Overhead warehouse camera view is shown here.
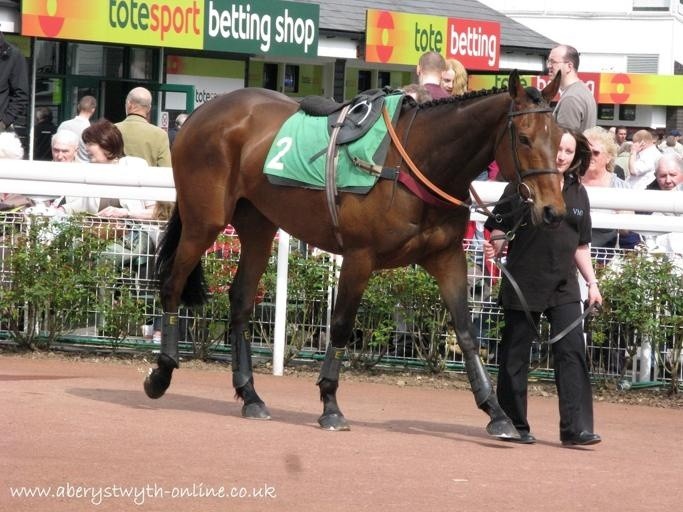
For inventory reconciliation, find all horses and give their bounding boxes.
[142,69,568,441]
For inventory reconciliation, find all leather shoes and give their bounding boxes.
[501,431,535,443]
[562,430,601,446]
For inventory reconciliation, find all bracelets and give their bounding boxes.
[585,279,601,288]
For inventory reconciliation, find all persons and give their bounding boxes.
[407,49,452,103]
[0,34,30,131]
[442,58,468,94]
[587,120,683,274]
[466,159,502,300]
[544,43,598,130]
[1,86,188,334]
[482,126,602,446]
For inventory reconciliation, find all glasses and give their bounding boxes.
[545,60,568,66]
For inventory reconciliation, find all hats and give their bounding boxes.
[671,129,681,137]
